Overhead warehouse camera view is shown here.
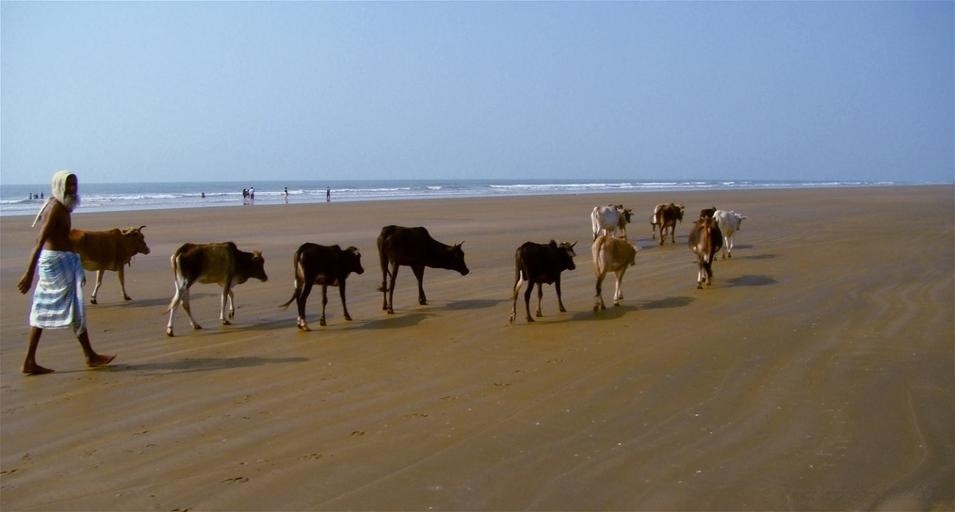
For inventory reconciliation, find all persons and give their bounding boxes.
[201,191,205,200]
[284,186,288,198]
[242,186,255,200]
[29,191,45,200]
[12,167,118,376]
[326,185,330,201]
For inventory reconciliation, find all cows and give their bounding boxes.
[688,207,748,290]
[591,235,639,313]
[509,240,578,322]
[590,204,635,242]
[161,242,268,337]
[278,242,365,332]
[68,225,151,305]
[649,203,685,246]
[376,225,469,315]
